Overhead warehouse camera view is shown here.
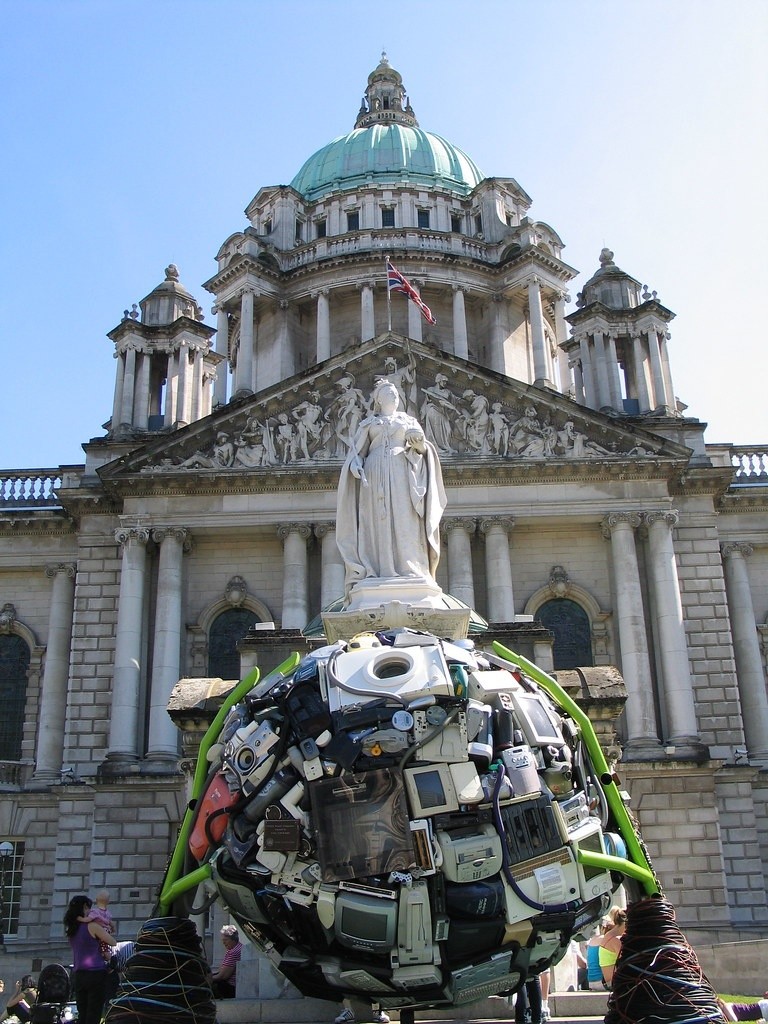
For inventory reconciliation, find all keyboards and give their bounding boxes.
[340,970,412,1009]
[453,972,520,1004]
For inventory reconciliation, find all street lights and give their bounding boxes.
[0,842,15,945]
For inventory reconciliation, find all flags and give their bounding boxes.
[387,261,436,326]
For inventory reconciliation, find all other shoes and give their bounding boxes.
[542,1007,551,1020]
[523,1007,533,1023]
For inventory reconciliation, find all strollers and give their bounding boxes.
[29,964,72,1024]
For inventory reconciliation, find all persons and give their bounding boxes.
[336,377,447,592]
[78,890,116,956]
[169,337,618,469]
[210,924,243,999]
[63,895,117,1024]
[514,904,627,1024]
[0,974,38,1024]
[717,998,768,1024]
[335,999,390,1024]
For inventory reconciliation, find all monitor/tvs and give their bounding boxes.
[508,692,565,748]
[403,763,459,820]
[309,766,419,884]
[574,740,587,799]
[214,877,269,924]
[258,889,329,956]
[335,892,398,954]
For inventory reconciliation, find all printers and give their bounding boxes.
[466,698,492,773]
[435,823,503,883]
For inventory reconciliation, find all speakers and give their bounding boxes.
[500,744,542,798]
[225,719,280,787]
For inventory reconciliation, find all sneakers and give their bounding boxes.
[335,1009,355,1023]
[372,1011,390,1023]
